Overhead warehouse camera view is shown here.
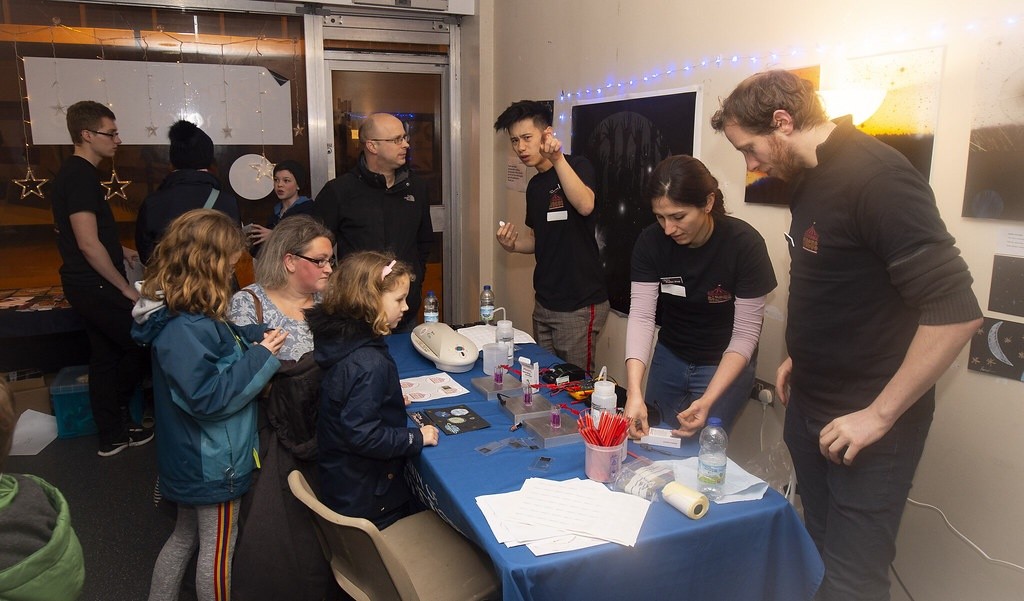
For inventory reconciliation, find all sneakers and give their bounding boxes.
[97,427,154,457]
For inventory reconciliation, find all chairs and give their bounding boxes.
[288,470,502,601]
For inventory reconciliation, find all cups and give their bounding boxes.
[482,342,509,376]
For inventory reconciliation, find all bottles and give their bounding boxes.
[696,416,729,502]
[480,285,495,326]
[590,380,617,430]
[423,290,439,323]
[495,320,515,367]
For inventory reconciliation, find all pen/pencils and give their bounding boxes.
[577,408,634,447]
[628,451,672,476]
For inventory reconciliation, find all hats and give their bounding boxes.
[168,120,215,171]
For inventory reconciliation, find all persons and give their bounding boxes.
[303,250,439,531]
[131,209,289,601]
[52,101,155,456]
[314,113,432,334]
[245,160,314,259]
[622,155,778,444]
[711,70,984,601]
[0,375,86,601]
[134,120,240,309]
[494,100,610,378]
[227,213,335,363]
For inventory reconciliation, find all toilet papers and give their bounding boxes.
[660,481,710,521]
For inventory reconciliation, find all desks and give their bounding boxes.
[381,322,826,601]
[0,287,86,337]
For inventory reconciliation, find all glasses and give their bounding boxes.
[292,253,334,267]
[85,128,119,140]
[371,133,410,145]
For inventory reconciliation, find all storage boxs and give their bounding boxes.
[3,364,145,440]
